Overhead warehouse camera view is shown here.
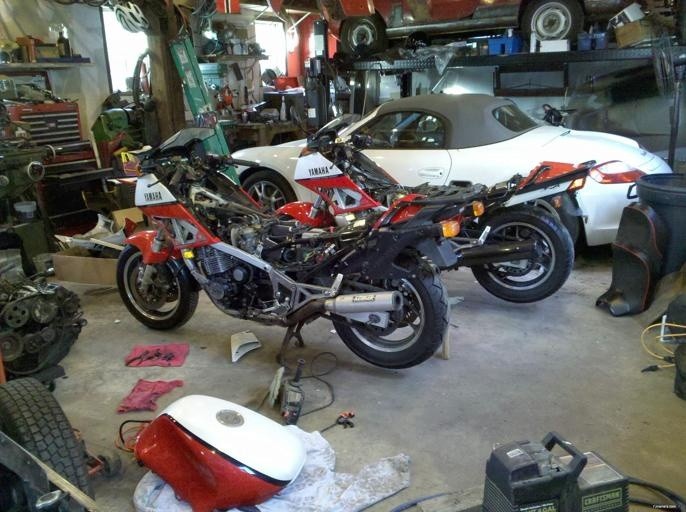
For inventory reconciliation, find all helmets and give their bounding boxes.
[406,31,431,49]
[114,0,150,33]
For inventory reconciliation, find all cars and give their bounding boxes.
[314,0,584,54]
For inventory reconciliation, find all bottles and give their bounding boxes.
[280,96,288,122]
[56,31,67,59]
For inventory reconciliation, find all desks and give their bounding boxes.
[219,117,307,154]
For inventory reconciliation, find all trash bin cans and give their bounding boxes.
[627,173,686,280]
